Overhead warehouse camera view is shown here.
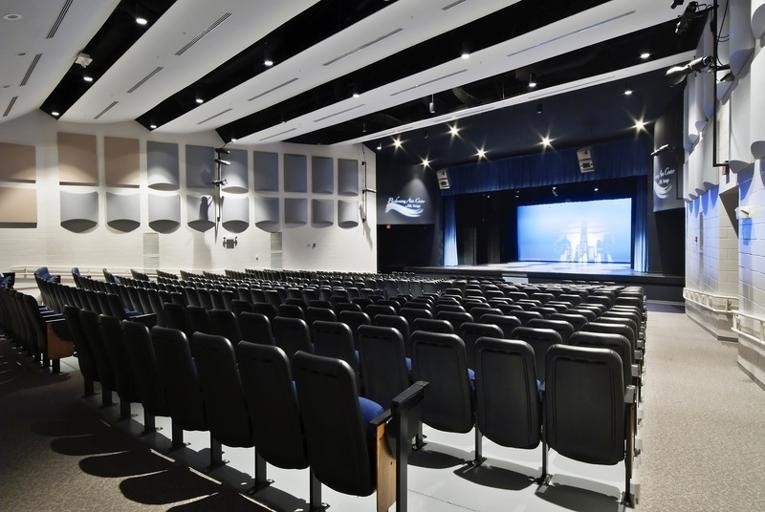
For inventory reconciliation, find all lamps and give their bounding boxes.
[212,148,230,187]
[651,142,676,159]
[665,55,713,88]
[671,0,713,36]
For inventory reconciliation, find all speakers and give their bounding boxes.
[578,143,604,173]
[437,170,454,188]
